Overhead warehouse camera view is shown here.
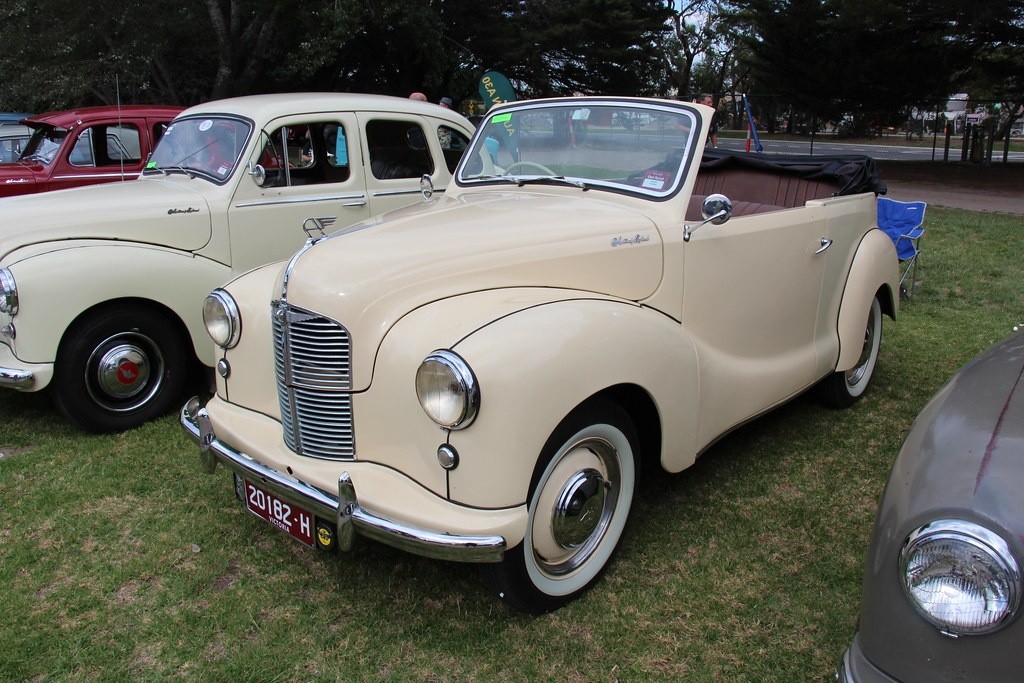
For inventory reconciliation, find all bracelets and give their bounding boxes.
[714,147,717,148]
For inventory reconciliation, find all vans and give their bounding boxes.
[927,93,973,135]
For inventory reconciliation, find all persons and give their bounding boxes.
[302,130,312,166]
[409,93,428,150]
[438,97,453,148]
[678,93,718,149]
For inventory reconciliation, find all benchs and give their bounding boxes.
[694,171,842,211]
[686,193,788,220]
[369,152,413,182]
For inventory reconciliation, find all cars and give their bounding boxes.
[1,120,142,164]
[838,328,1024,683]
[1010,129,1024,136]
[0,105,294,199]
[0,94,514,435]
[180,91,900,616]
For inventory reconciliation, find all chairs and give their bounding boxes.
[877,198,927,294]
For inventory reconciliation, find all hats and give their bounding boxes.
[440,97,452,107]
